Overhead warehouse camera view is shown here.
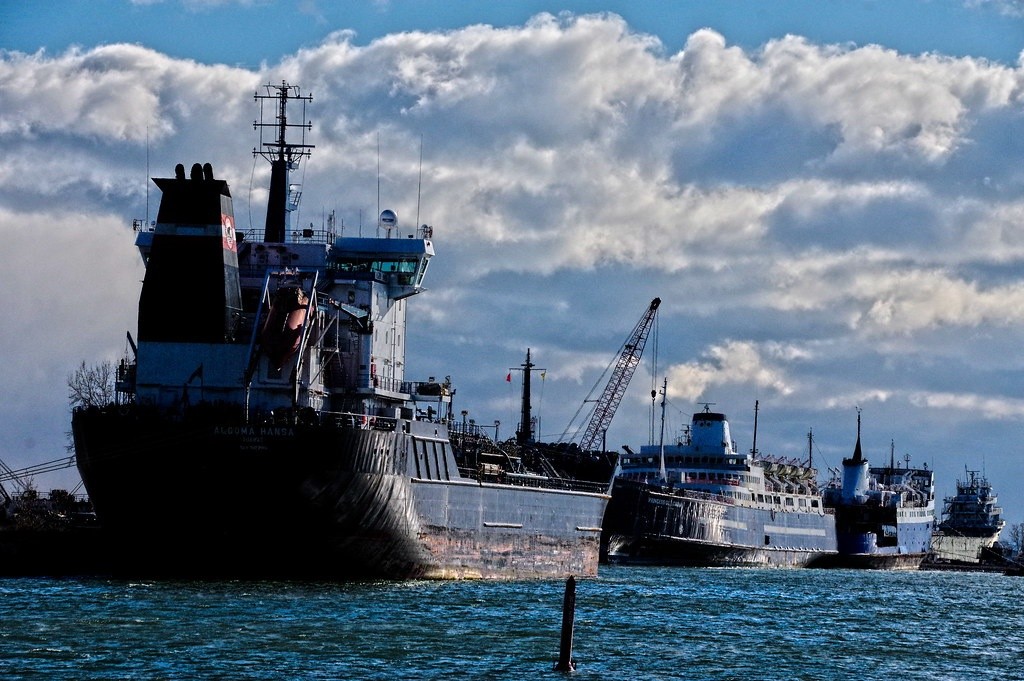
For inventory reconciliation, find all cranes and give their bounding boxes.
[563,294,659,474]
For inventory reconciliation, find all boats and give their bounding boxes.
[978,522,1024,574]
[926,462,1007,567]
[68,83,626,582]
[612,377,841,568]
[819,406,937,569]
